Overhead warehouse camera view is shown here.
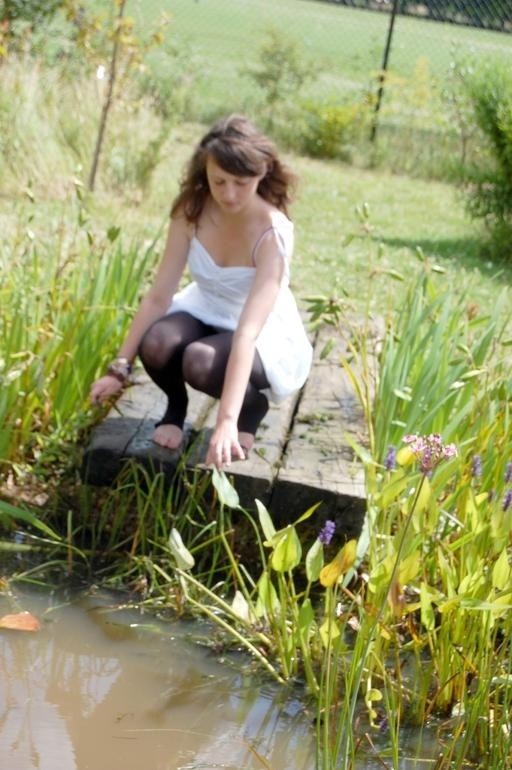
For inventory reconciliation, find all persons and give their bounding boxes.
[87,115,314,473]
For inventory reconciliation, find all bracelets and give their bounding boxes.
[102,354,134,380]
